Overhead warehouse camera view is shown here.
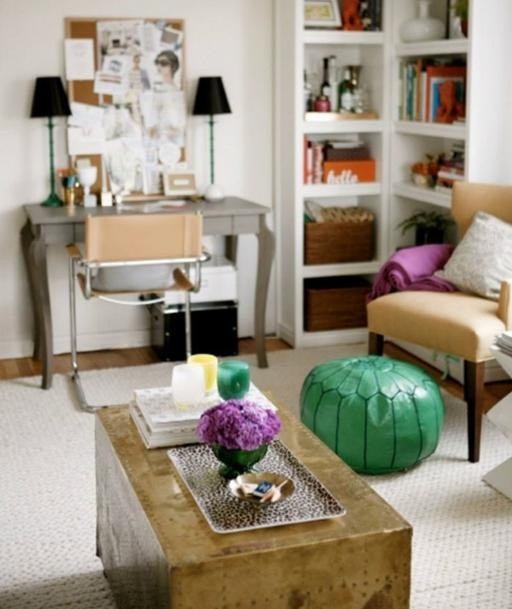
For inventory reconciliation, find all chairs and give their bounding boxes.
[67,207,203,378]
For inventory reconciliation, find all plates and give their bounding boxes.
[166,439,348,534]
[228,470,296,505]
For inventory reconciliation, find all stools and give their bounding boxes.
[301,355,446,474]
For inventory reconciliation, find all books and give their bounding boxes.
[128,379,278,449]
[400,57,466,124]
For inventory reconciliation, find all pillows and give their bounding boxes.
[431,211,511,300]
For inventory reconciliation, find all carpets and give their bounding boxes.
[0,350,512,609]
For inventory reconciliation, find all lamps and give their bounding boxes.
[30,76,73,208]
[190,77,233,200]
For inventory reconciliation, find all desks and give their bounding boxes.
[21,201,275,390]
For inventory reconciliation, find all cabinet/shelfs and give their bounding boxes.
[387,0,511,264]
[274,0,386,348]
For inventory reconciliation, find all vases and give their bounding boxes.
[211,442,270,479]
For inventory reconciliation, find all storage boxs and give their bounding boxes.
[305,219,375,265]
[323,157,375,183]
[304,280,371,332]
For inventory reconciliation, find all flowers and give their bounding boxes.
[199,401,282,452]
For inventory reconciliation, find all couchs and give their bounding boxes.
[368,179,511,465]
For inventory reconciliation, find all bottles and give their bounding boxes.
[319,57,331,111]
[338,69,354,116]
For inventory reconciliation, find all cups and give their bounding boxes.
[172,364,205,409]
[217,359,251,402]
[187,353,219,391]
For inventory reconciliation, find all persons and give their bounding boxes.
[127,54,150,90]
[436,81,465,123]
[154,50,179,90]
